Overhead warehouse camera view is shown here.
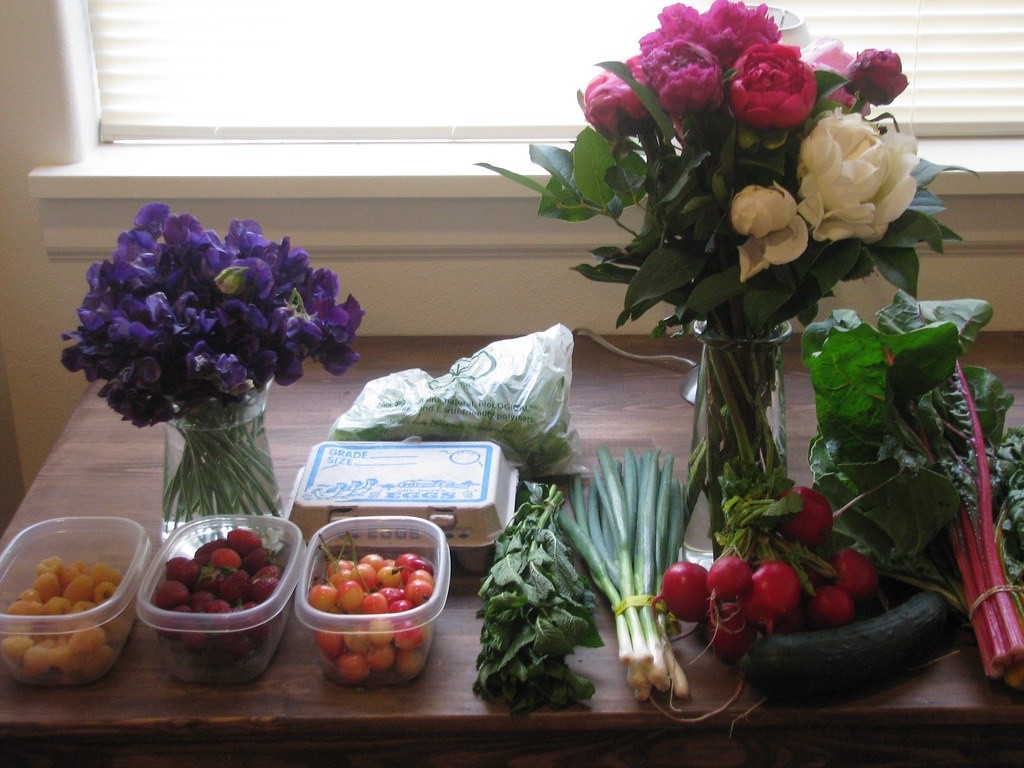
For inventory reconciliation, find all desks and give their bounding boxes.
[0,333,1023,768]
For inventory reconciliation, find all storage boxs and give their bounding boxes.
[0,515,152,688]
[137,516,306,684]
[294,514,451,685]
[289,441,520,570]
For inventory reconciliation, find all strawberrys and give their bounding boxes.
[151,528,285,669]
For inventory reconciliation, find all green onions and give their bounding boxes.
[558,446,691,701]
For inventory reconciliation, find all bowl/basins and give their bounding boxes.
[136,513,309,687]
[294,515,451,688]
[0,516,152,688]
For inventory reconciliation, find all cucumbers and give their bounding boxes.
[735,588,954,694]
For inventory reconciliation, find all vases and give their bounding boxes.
[162,374,277,544]
[677,319,793,568]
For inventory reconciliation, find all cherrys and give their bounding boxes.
[312,530,434,685]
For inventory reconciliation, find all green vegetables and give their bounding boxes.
[334,342,575,476]
[795,290,1024,690]
[472,478,605,726]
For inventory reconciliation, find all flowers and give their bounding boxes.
[60,201,365,520]
[477,0,980,567]
